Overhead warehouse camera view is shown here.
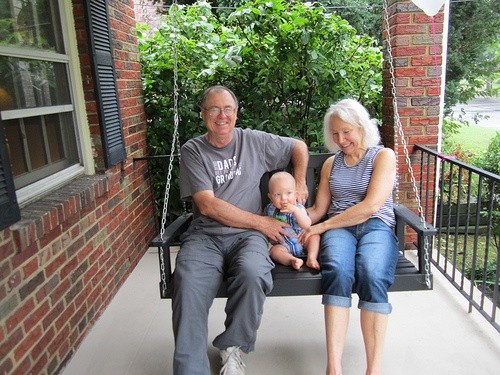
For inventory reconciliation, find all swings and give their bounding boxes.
[147,0,438,299]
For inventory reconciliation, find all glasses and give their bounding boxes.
[199,105,236,117]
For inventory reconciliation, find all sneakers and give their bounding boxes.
[217,345,246,374]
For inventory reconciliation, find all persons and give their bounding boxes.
[262,172,320,270]
[171,85,308,375]
[296,98,399,375]
